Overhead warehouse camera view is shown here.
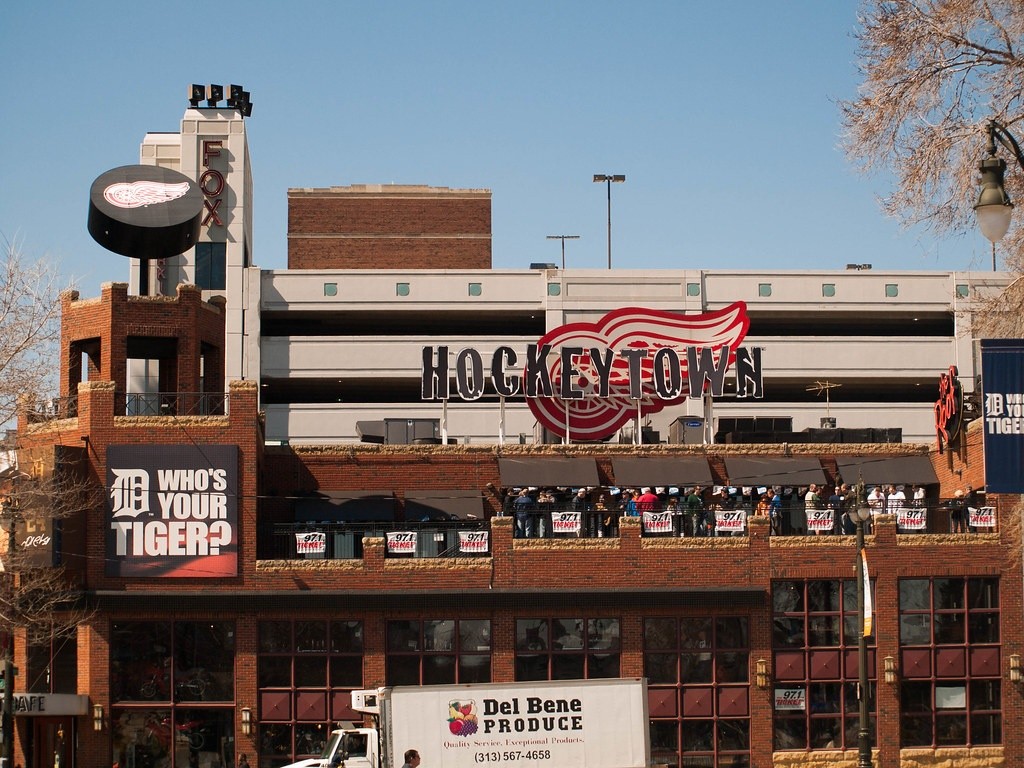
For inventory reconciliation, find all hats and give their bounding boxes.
[721,487,729,494]
[772,485,782,495]
[966,485,973,491]
[621,489,629,493]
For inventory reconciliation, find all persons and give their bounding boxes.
[402,749,421,768]
[504,483,978,535]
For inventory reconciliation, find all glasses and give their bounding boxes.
[813,488,816,490]
[875,489,881,492]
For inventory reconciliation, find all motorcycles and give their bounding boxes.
[141,657,207,700]
[141,713,207,750]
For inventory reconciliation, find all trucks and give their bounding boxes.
[277,676,653,768]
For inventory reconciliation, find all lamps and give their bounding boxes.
[884,653,895,684]
[756,656,766,687]
[240,707,252,735]
[1010,652,1021,682]
[94,703,103,731]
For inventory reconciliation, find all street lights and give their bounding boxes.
[593,173,626,270]
[546,234,580,268]
[843,480,873,768]
